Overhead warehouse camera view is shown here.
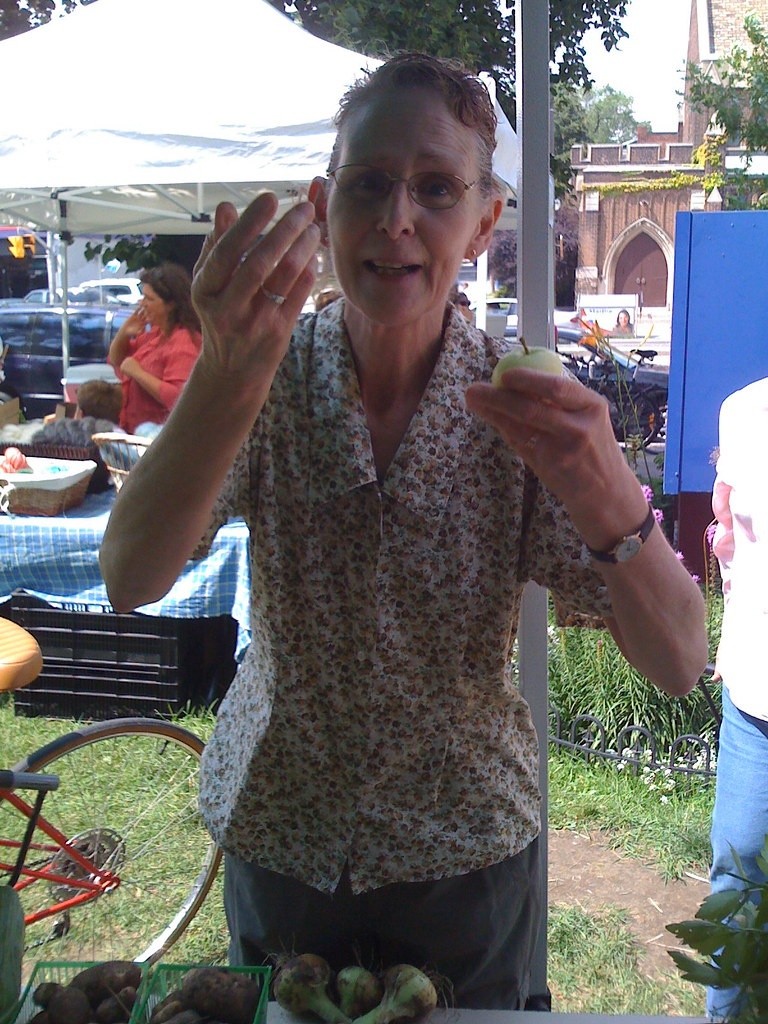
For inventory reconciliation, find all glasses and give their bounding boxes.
[457,301,471,306]
[327,162,484,210]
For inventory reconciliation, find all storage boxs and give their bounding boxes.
[10,961,272,1024]
[10,591,204,722]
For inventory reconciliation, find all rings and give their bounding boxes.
[260,282,286,305]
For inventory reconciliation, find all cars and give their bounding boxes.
[23,278,145,304]
[505,329,669,441]
[0,304,139,421]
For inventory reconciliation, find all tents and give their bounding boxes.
[0,0,555,376]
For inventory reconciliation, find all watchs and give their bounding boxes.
[586,504,655,565]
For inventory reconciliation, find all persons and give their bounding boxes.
[692,377,768,1018]
[108,262,203,433]
[613,310,633,334]
[450,281,476,326]
[99,52,709,1012]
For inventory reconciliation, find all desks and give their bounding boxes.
[0,488,251,670]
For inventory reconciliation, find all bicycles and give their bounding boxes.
[555,348,663,451]
[0,617,223,967]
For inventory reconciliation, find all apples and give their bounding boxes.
[490,335,562,386]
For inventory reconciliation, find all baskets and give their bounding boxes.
[12,960,149,1023]
[135,963,272,1024]
[0,442,110,479]
[92,432,156,493]
[0,456,97,517]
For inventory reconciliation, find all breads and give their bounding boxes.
[0,415,114,448]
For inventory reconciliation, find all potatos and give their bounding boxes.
[22,959,263,1024]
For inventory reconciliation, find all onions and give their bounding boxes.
[269,949,439,1024]
